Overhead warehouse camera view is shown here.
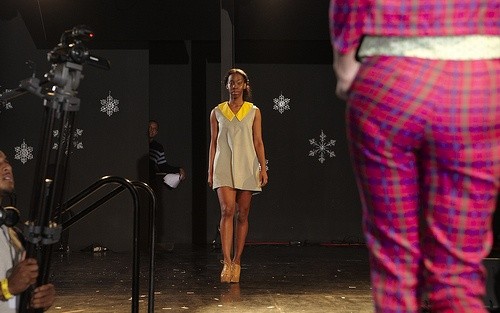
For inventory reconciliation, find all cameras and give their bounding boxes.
[48,28,110,67]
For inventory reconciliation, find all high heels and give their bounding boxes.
[220,263,231,282]
[230,263,241,282]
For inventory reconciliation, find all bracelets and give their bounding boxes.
[1,277,14,300]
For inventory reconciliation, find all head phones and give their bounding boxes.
[0,206,20,228]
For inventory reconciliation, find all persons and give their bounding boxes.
[148,120,187,249]
[0,150,57,313]
[208,68,269,283]
[330,0,500,313]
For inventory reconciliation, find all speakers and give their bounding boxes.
[480,257,500,313]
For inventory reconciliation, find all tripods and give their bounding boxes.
[0,62,81,313]
